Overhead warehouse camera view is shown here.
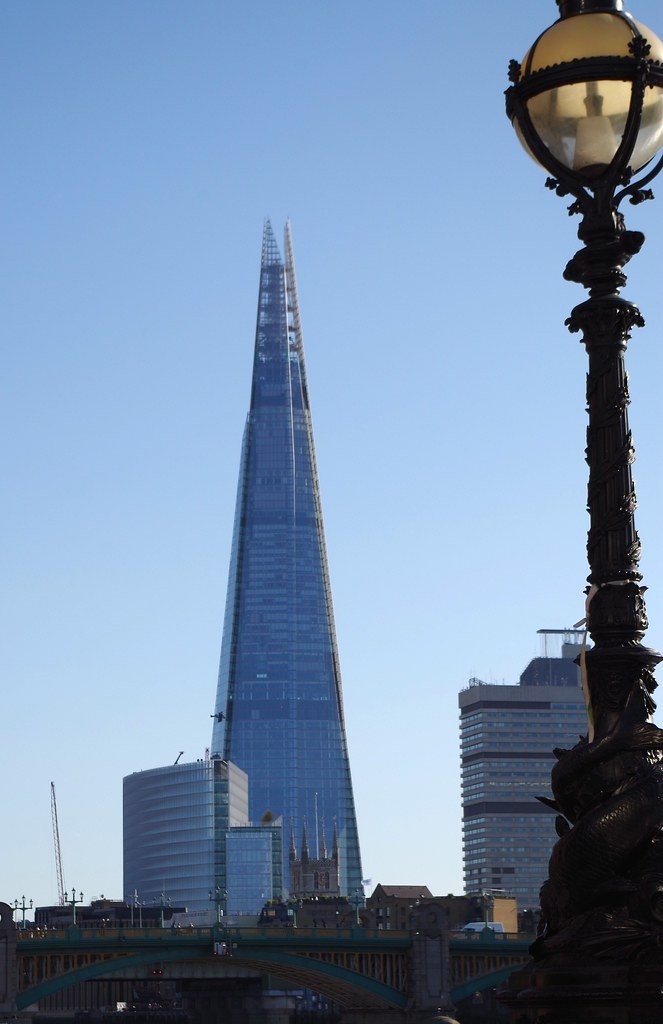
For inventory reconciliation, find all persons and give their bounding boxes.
[122,1004,130,1012]
[336,923,339,929]
[99,919,111,936]
[171,921,195,936]
[287,921,297,937]
[29,925,56,939]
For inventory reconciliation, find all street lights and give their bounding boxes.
[208,886,228,922]
[502,1,663,1015]
[14,894,33,931]
[64,887,84,925]
[153,893,172,928]
